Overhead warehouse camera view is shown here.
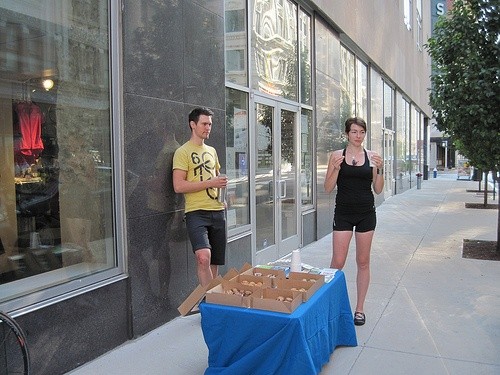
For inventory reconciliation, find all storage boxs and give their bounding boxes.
[269,278,317,302]
[285,272,325,290]
[253,288,303,314]
[177,278,264,317]
[232,262,286,288]
[216,267,272,289]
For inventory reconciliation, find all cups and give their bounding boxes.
[291,250,301,271]
[219,174,227,203]
[369,151,378,167]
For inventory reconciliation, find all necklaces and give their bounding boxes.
[348,147,361,159]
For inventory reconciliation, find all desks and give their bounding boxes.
[198,265,359,375]
[15,176,49,251]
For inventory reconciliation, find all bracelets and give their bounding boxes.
[377,167,384,175]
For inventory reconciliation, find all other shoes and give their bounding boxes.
[135,294,171,316]
[354,311,365,325]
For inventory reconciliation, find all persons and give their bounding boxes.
[325,117,384,325]
[173,106,228,288]
